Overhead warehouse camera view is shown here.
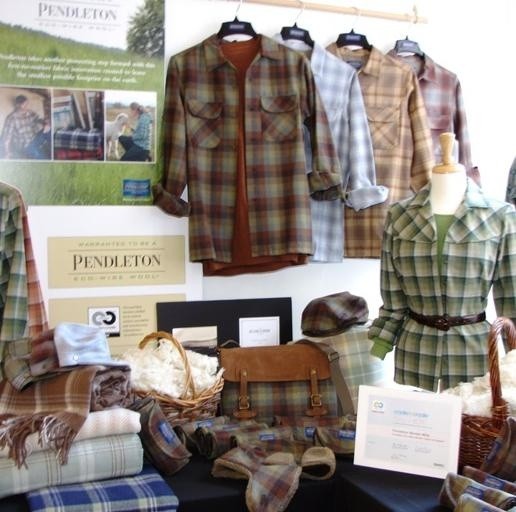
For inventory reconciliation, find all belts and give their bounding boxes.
[407,309,486,331]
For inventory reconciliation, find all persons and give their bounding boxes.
[3,91,49,158]
[366,162,516,395]
[118,100,153,162]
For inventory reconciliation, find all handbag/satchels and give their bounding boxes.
[220,346,338,420]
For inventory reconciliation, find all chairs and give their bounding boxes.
[156,296,294,353]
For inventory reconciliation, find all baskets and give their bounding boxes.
[458,317,516,471]
[132,333,225,426]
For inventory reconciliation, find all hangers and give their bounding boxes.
[325,4,382,60]
[212,0,265,44]
[269,0,320,57]
[387,13,428,63]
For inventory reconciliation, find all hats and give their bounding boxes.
[211,439,336,511]
[302,292,369,337]
